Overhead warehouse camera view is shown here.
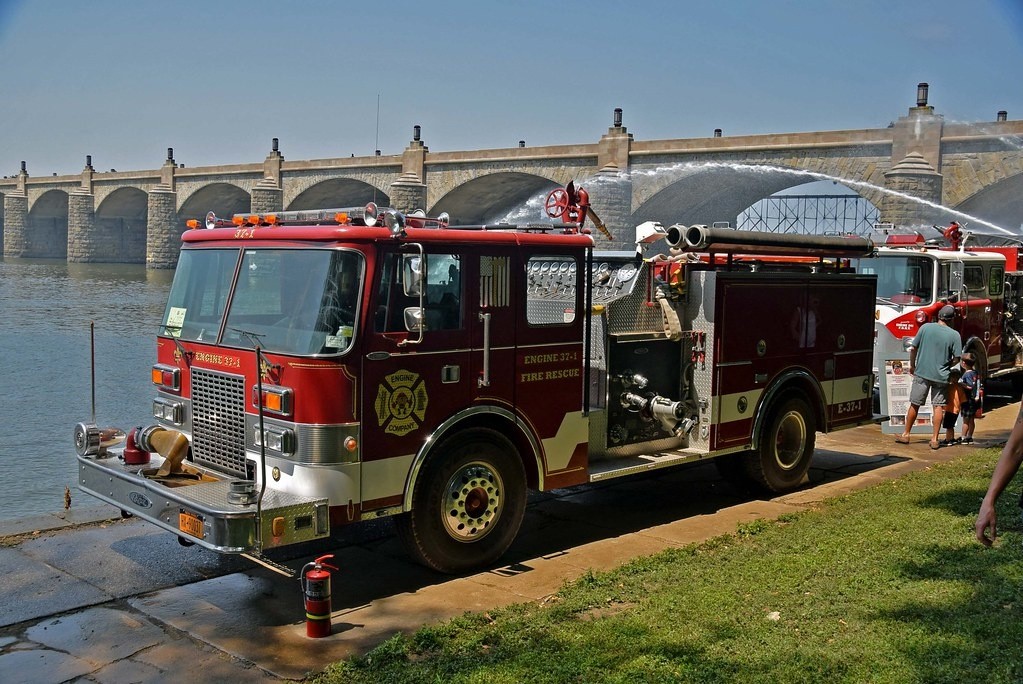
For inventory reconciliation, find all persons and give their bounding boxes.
[956,353,980,445]
[894,305,962,448]
[975,395,1023,547]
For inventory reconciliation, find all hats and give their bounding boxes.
[938,305,955,320]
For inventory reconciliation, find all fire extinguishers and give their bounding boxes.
[300,554,340,639]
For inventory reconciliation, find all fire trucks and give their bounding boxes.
[73,175,877,577]
[834,224,1023,409]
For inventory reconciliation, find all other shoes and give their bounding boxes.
[939,439,952,445]
[895,435,910,443]
[951,439,957,444]
[967,439,974,444]
[929,441,939,449]
[956,437,968,444]
